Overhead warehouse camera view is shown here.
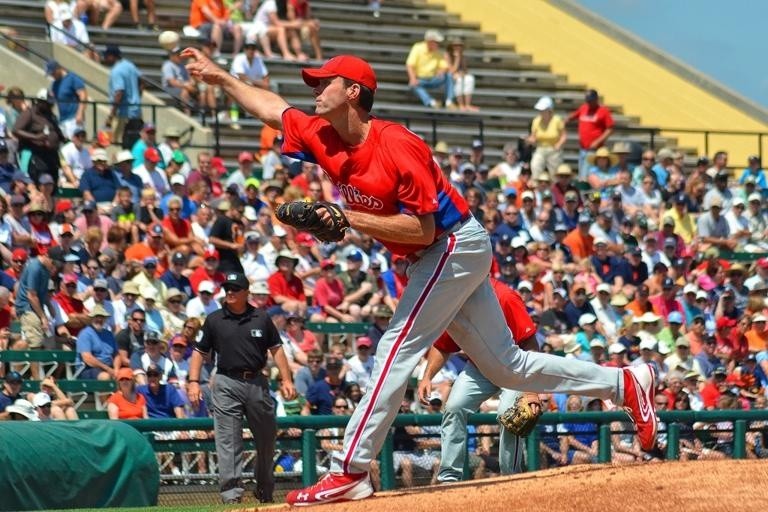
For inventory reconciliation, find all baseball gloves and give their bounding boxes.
[275,201,350,242]
[499,392,543,437]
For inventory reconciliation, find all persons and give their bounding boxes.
[1,0,768,508]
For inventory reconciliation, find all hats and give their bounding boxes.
[533,97,554,110]
[170,39,257,54]
[585,90,597,102]
[36,88,57,105]
[46,60,57,75]
[302,56,377,91]
[424,29,444,42]
[0,123,407,422]
[427,138,768,410]
[103,46,121,57]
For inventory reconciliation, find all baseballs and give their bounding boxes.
[159,30,179,49]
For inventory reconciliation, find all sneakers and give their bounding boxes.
[286,472,375,507]
[621,364,659,450]
